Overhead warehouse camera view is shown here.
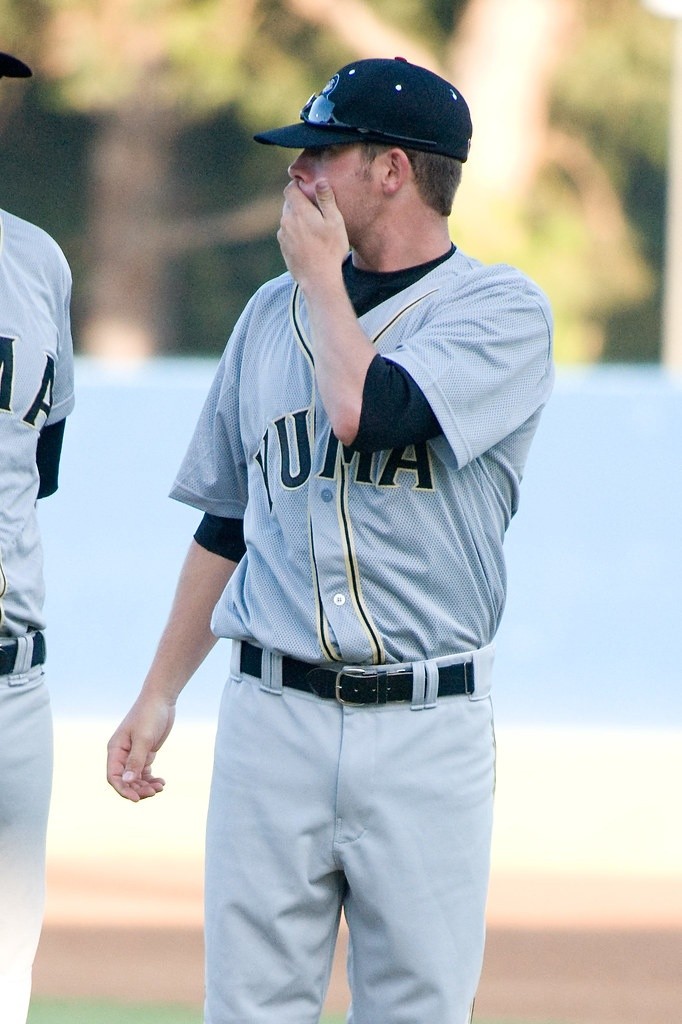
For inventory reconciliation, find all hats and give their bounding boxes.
[253,57,472,163]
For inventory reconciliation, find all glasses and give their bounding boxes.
[300,92,436,146]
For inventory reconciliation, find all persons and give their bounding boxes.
[107,56,554,1024]
[0,47,74,1024]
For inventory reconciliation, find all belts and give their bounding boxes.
[240,640,474,707]
[0,632,45,677]
